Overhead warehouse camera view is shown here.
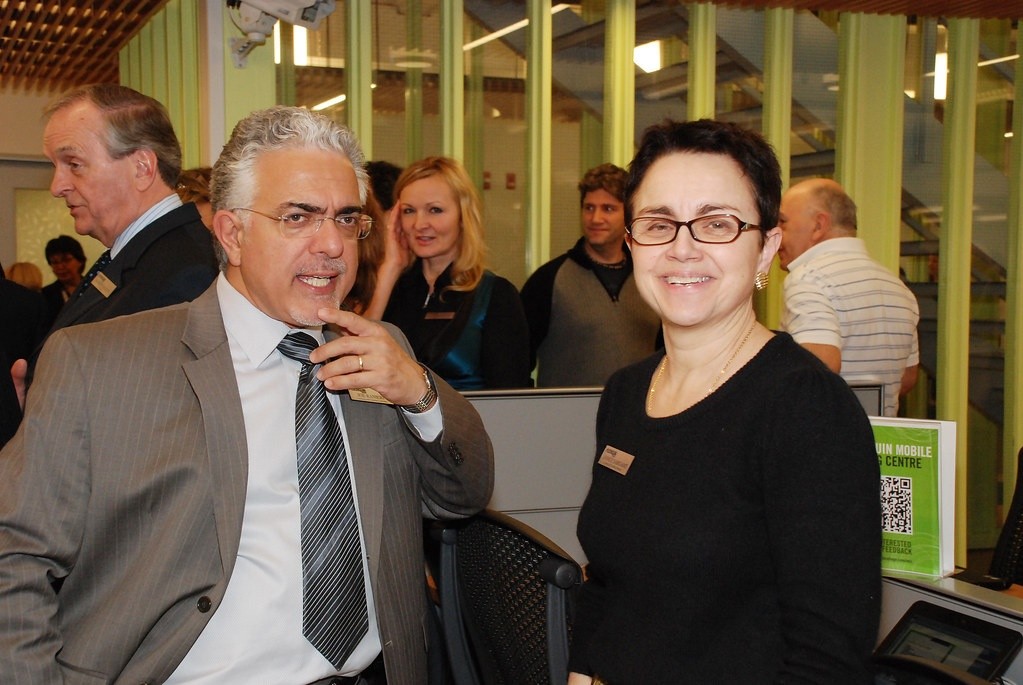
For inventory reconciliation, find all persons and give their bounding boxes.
[521,164,660,389]
[0,104,495,685]
[777,177,921,416]
[44,84,221,330]
[0,259,53,450]
[568,118,883,685]
[365,156,529,392]
[42,234,87,305]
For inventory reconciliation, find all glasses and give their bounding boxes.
[229,208,376,241]
[50,256,76,266]
[624,214,766,247]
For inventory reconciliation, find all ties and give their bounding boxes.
[71,252,111,305]
[277,333,372,675]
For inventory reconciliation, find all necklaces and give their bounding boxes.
[648,321,756,414]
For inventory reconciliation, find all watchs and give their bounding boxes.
[404,368,436,413]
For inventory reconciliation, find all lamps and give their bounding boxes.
[933,25,950,106]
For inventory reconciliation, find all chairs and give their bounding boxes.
[439,511,578,685]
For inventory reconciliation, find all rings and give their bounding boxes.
[358,356,363,370]
